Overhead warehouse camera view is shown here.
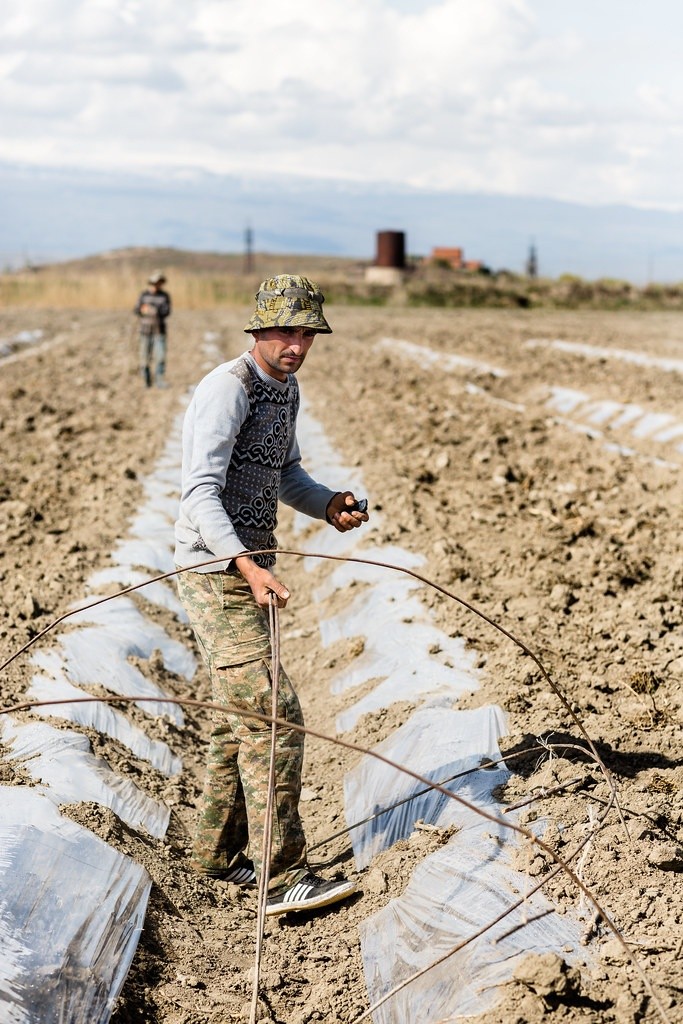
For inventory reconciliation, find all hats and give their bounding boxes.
[147,273,165,285]
[244,275,334,334]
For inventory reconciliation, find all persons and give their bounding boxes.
[133,274,171,389]
[173,274,370,916]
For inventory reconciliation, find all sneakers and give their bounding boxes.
[198,852,259,888]
[265,872,357,916]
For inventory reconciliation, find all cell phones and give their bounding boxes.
[331,499,367,520]
